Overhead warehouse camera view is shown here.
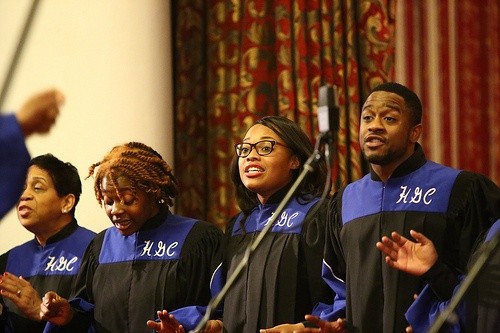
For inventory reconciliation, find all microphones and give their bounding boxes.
[316,86,338,166]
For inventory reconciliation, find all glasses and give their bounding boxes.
[234,139,298,157]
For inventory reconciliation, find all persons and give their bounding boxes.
[40,141,224,333]
[0,87,65,218]
[292,82,500,332]
[201,115,330,333]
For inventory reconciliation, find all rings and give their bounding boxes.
[17,289,22,296]
[0,152,100,333]
